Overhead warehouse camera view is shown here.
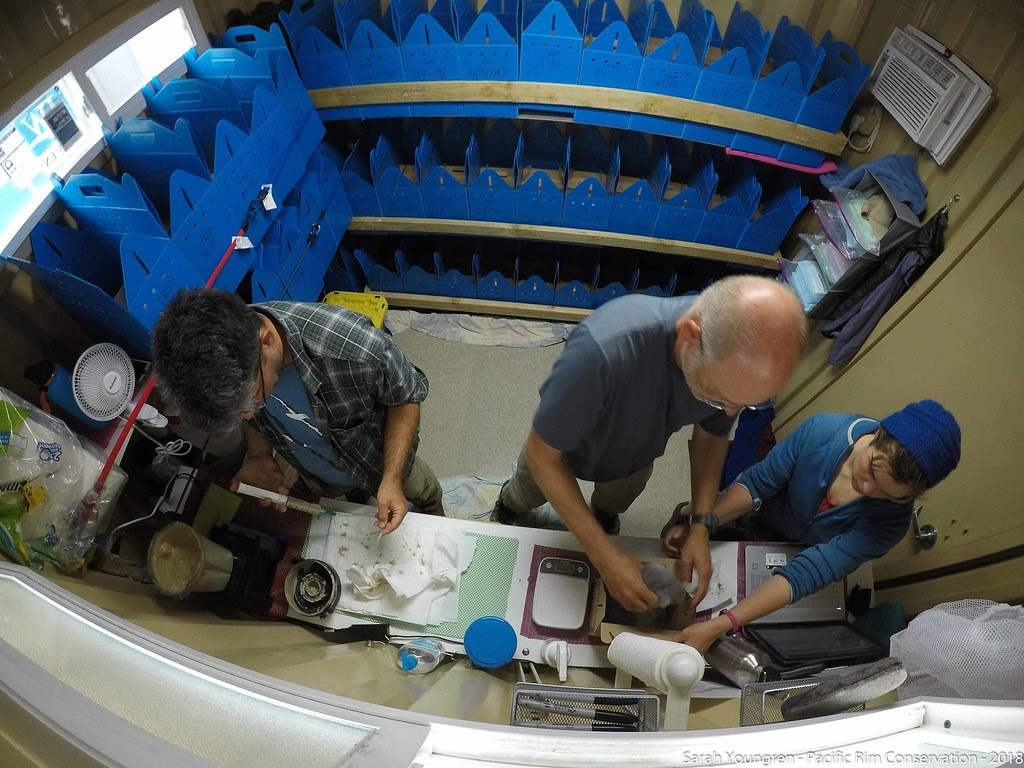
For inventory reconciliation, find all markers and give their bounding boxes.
[513,691,640,731]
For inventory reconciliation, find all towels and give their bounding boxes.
[819,154,930,217]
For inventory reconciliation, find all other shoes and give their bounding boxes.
[595,509,620,535]
[425,500,445,516]
[490,479,520,525]
[661,502,690,538]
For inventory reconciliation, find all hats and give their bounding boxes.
[880,399,961,489]
[861,194,894,240]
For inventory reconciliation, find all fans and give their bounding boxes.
[71,343,135,422]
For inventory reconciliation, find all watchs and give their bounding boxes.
[689,512,719,534]
[719,608,740,637]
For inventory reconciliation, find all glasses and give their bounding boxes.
[692,309,776,412]
[240,326,267,423]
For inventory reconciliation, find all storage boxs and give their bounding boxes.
[0,0,872,360]
[586,553,698,645]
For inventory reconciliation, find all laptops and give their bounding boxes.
[744,545,890,664]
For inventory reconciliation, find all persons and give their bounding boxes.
[153,284,445,534]
[490,274,810,613]
[660,400,963,656]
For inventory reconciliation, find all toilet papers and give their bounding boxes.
[2,389,122,574]
[607,631,706,697]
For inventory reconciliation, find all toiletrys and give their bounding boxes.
[394,640,445,671]
